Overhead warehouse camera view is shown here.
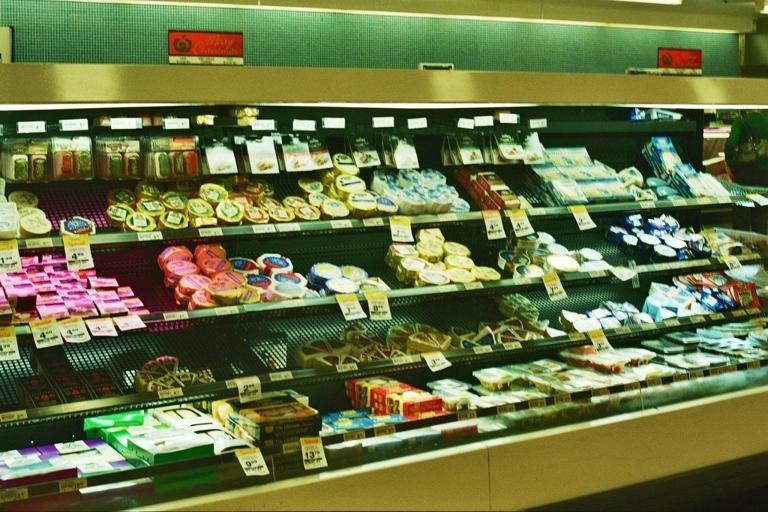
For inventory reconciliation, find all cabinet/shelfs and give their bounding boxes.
[0,61,768,511]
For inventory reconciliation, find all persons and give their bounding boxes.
[723,123,767,257]
